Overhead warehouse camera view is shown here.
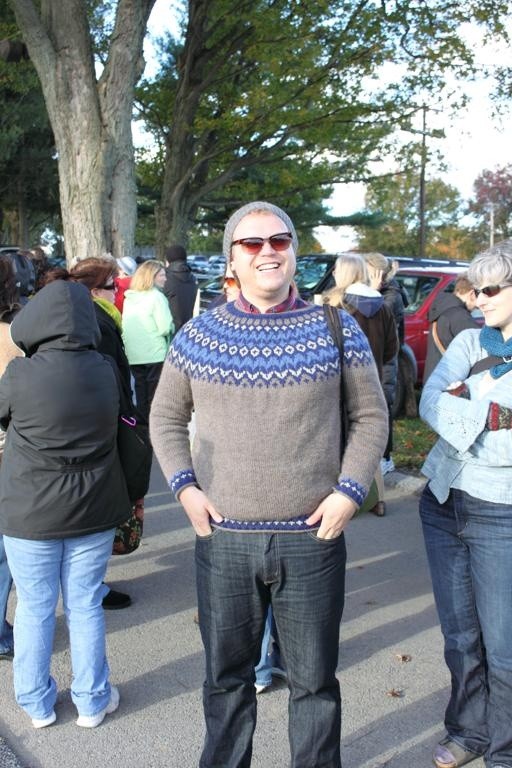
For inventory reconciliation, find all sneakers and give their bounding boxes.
[369,501,385,516]
[380,455,395,476]
[76,685,120,728]
[30,705,57,729]
[0,648,14,661]
[255,653,290,694]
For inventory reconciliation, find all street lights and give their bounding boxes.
[485,202,500,248]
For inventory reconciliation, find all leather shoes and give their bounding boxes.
[102,588,132,610]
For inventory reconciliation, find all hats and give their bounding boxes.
[222,201,298,262]
[165,245,186,263]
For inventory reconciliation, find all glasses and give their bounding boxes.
[473,283,511,299]
[231,233,294,255]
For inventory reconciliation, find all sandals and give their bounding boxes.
[432,735,483,768]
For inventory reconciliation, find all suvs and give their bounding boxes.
[384,267,484,420]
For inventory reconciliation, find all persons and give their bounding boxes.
[222,261,289,692]
[0,280,133,726]
[148,201,390,768]
[361,252,409,475]
[421,273,483,390]
[309,252,400,517]
[1,247,200,661]
[418,240,512,768]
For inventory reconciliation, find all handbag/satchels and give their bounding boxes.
[110,510,142,555]
[117,412,152,504]
